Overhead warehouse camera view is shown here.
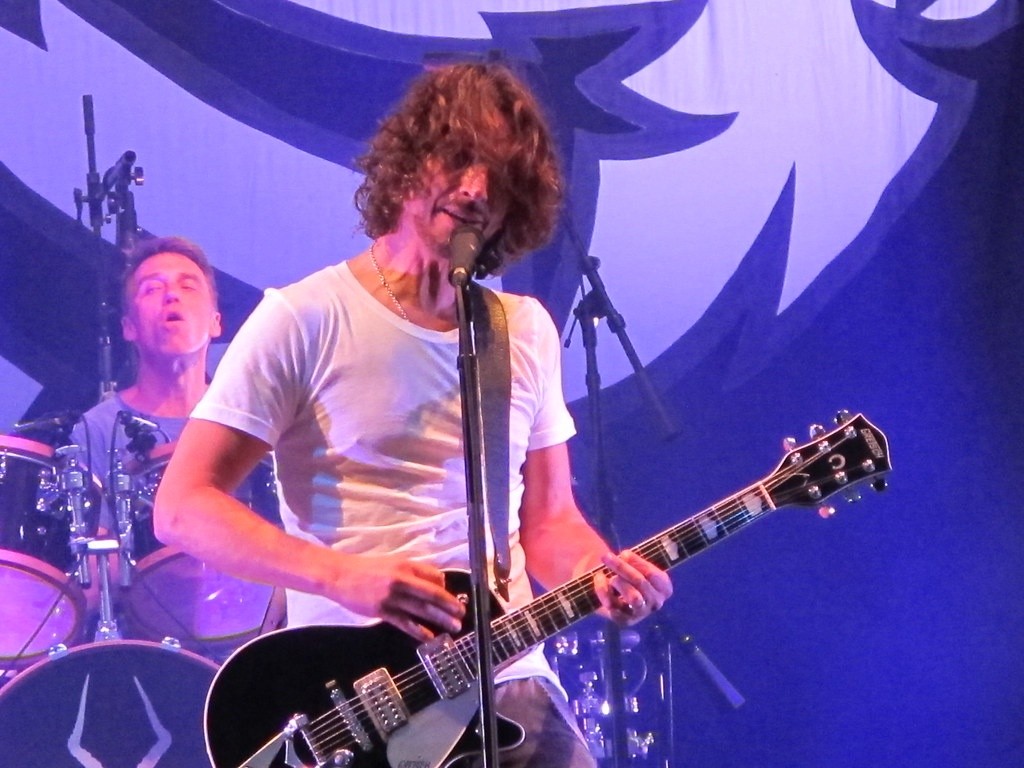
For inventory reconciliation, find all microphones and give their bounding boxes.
[448,223,487,286]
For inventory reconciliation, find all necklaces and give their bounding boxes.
[371,239,413,323]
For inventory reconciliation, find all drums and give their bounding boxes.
[0,630,226,768]
[114,447,295,649]
[0,435,88,669]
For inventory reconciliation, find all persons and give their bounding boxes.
[150,58,673,768]
[58,234,290,668]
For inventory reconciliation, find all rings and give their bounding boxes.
[628,599,646,610]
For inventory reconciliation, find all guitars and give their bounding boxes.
[202,411,897,767]
